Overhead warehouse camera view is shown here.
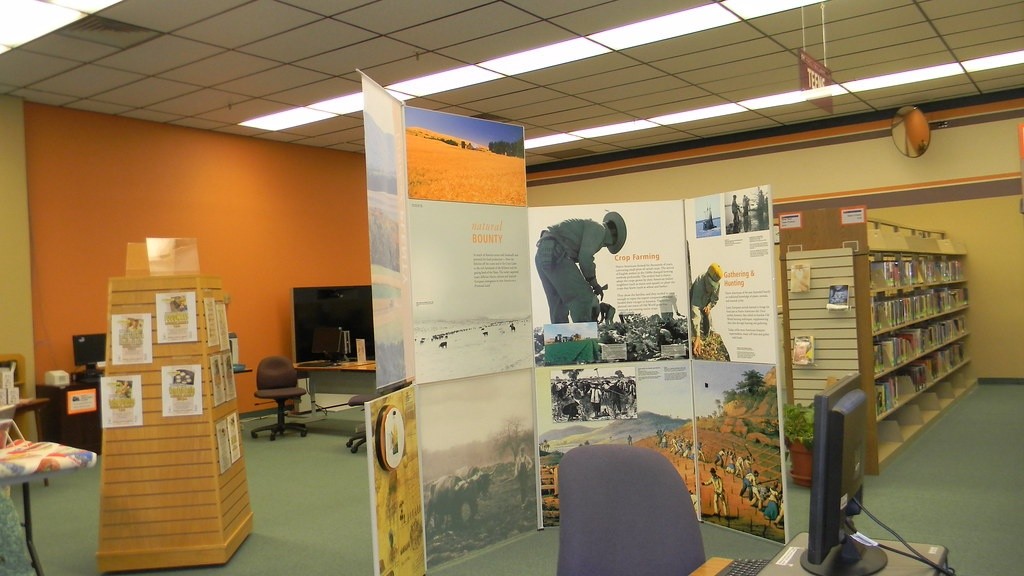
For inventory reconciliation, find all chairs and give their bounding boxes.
[346,394,377,453]
[251,355,309,440]
[558,444,705,576]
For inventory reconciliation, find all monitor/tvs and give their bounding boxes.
[799,371,888,576]
[310,325,343,363]
[72,333,106,375]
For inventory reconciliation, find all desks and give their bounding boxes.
[0,405,97,576]
[0,353,52,486]
[292,360,376,425]
[690,531,950,576]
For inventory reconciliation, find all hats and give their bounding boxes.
[603,212,627,254]
[710,468,716,473]
[706,263,723,288]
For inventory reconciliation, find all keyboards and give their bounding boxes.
[714,557,771,576]
[297,362,333,367]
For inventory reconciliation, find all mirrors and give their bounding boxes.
[892,105,930,158]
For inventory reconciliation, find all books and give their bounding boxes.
[203,296,241,472]
[870,258,969,417]
[99,291,202,428]
[829,285,849,306]
[792,263,810,293]
[794,336,814,365]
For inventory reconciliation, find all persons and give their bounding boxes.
[535,212,627,324]
[555,334,579,342]
[731,187,769,224]
[552,378,636,421]
[515,446,532,510]
[691,264,721,356]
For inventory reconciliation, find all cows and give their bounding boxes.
[425,464,498,531]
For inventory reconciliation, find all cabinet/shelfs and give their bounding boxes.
[778,206,980,475]
[36,380,100,456]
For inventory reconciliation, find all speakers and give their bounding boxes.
[341,331,352,361]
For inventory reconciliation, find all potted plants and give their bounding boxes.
[782,403,816,486]
